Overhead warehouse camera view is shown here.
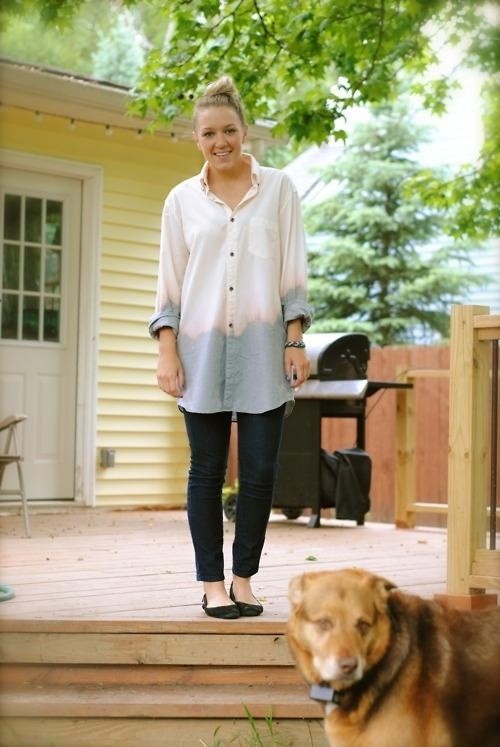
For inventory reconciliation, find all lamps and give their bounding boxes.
[0,100,178,143]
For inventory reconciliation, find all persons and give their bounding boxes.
[146,75,313,620]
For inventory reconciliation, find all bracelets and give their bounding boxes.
[284,341,308,347]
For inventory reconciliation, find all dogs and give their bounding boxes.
[282,567,500,747]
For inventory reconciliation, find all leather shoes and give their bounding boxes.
[230,581,263,616]
[202,594,240,619]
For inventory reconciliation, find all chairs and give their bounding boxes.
[0,412,30,539]
